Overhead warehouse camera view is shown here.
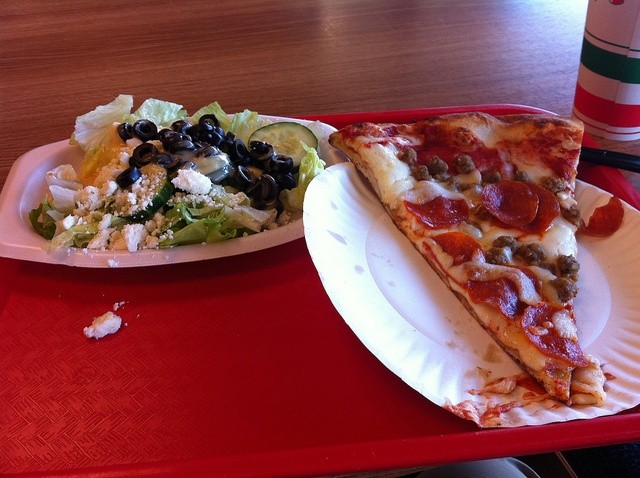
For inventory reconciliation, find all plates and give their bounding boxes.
[302,161,640,429]
[0,114,353,268]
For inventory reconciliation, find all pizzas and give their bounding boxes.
[329,112,607,407]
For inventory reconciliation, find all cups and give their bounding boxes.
[572,0,640,140]
